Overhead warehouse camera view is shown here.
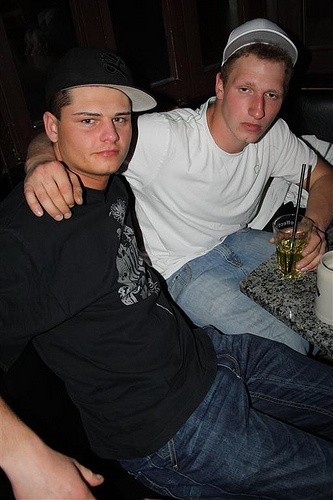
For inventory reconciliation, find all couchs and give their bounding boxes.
[158,83,333,234]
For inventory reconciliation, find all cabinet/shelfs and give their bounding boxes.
[0,0,192,201]
[266,0,333,94]
[182,0,268,99]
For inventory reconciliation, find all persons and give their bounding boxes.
[0,58,333,499]
[21,8,75,106]
[24,16,333,361]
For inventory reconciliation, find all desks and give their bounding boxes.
[238,222,333,358]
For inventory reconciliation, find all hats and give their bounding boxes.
[221,18,297,69]
[45,45,157,114]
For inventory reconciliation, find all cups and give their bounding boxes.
[313,251,333,325]
[272,214,313,280]
